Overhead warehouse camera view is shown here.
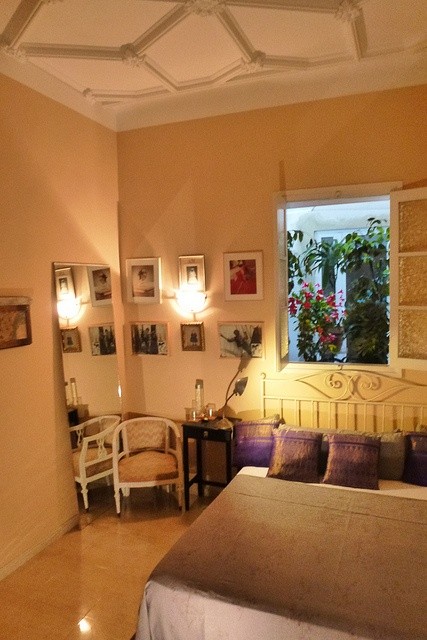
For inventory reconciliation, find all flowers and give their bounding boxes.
[287,280,349,363]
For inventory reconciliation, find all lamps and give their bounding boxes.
[214,376,247,429]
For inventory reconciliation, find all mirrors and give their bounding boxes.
[52,261,124,532]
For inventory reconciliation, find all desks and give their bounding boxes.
[181,417,243,514]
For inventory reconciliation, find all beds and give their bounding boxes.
[132,369,427,639]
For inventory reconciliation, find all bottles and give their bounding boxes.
[194,384,205,417]
[70,378,78,407]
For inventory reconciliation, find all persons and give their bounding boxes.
[98,329,114,355]
[230,260,256,294]
[133,268,154,297]
[220,329,253,355]
[135,327,159,355]
[94,273,112,300]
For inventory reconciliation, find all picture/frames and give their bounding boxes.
[180,322,205,352]
[0,304,32,351]
[88,323,115,356]
[222,251,264,300]
[130,320,169,355]
[126,258,162,304]
[177,254,205,292]
[60,326,81,352]
[87,265,113,307]
[55,267,76,299]
[216,321,264,359]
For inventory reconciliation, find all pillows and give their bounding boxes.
[334,430,404,482]
[266,425,323,482]
[400,431,427,486]
[234,414,283,466]
[279,424,335,453]
[328,434,382,491]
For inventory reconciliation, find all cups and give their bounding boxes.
[185,408,196,422]
[206,404,216,421]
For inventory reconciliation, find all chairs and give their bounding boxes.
[112,418,185,516]
[67,416,122,511]
